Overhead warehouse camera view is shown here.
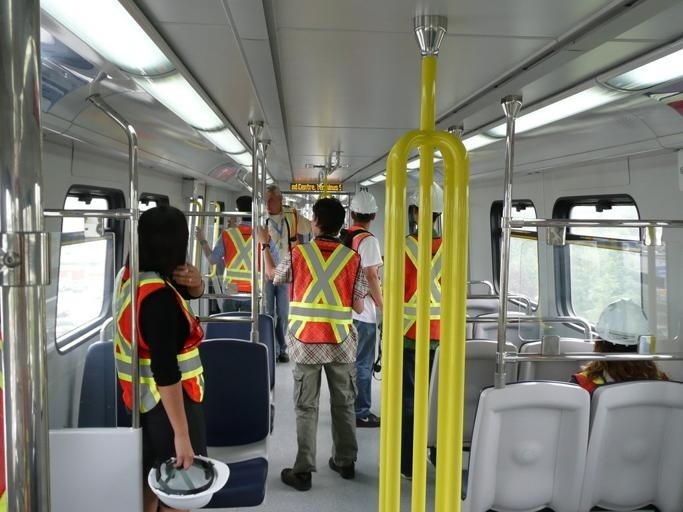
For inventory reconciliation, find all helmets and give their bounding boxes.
[596,299,650,345]
[349,192,378,214]
[148,455,230,510]
[408,181,442,213]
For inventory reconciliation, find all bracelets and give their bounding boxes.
[260,244,270,250]
[199,240,207,246]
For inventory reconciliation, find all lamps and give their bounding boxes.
[40,0,276,190]
[355,33,682,191]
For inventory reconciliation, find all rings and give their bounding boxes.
[188,278,191,284]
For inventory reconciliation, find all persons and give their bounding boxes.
[338,191,383,427]
[568,297,670,391]
[194,196,261,314]
[257,198,369,492]
[113,206,204,509]
[262,185,312,364]
[401,182,448,481]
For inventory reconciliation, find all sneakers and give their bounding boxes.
[356,414,380,427]
[281,468,311,490]
[329,457,355,479]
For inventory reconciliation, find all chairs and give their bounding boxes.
[423,278,683,512]
[76,311,276,510]
